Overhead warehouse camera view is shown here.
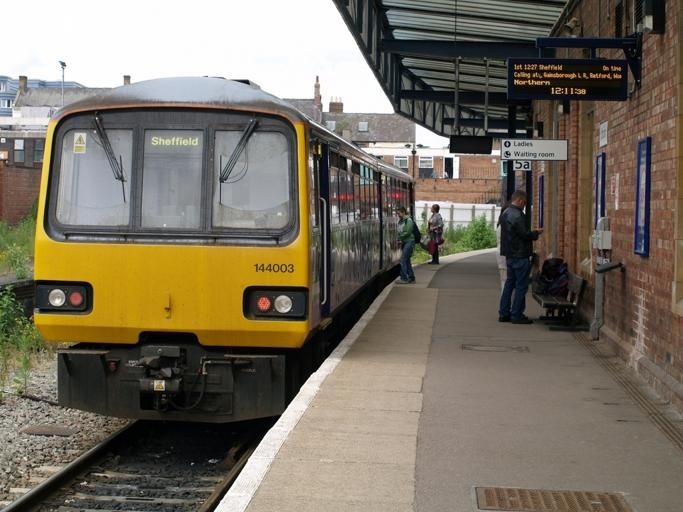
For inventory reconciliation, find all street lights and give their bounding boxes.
[403,143,422,176]
[55,60,69,109]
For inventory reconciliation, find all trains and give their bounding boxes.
[30,75,415,426]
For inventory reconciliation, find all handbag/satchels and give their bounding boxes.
[420,235,445,255]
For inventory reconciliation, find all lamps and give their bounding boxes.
[563,17,581,31]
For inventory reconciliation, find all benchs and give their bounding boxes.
[532,272,589,331]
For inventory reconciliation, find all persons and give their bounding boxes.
[395,206,416,283]
[497,189,542,324]
[426,203,442,264]
[495,204,509,296]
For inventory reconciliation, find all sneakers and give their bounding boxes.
[406,278,416,284]
[499,315,512,323]
[428,261,439,265]
[395,279,409,284]
[511,316,533,325]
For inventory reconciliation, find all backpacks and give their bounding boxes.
[404,218,421,243]
[532,257,568,295]
